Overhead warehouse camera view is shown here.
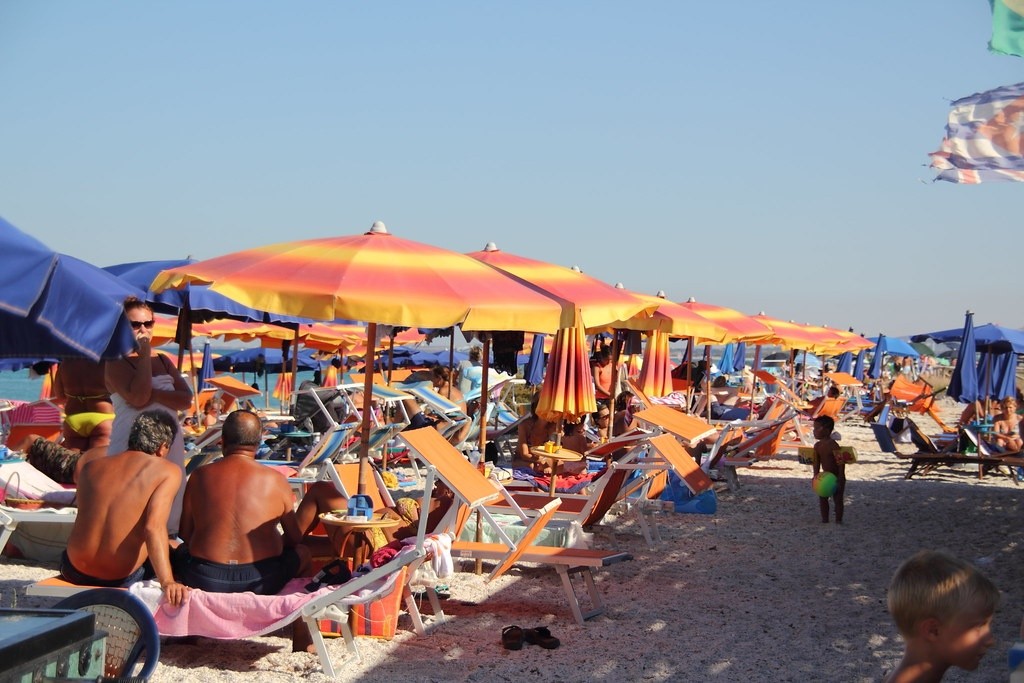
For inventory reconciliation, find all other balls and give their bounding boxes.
[812,471,838,497]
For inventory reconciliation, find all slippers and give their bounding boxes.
[500,625,526,650]
[523,626,561,650]
[409,583,451,600]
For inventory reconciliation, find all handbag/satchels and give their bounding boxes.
[484,442,499,466]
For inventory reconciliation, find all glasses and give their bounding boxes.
[598,413,610,420]
[130,321,155,330]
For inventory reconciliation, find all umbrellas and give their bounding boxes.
[0,215,1023,480]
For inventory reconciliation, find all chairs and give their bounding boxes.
[0,372,1024,683]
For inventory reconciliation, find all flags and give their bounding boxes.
[923,0,1024,188]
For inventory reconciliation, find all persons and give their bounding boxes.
[13,294,1024,683]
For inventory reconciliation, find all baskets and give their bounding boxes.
[6,498,47,510]
